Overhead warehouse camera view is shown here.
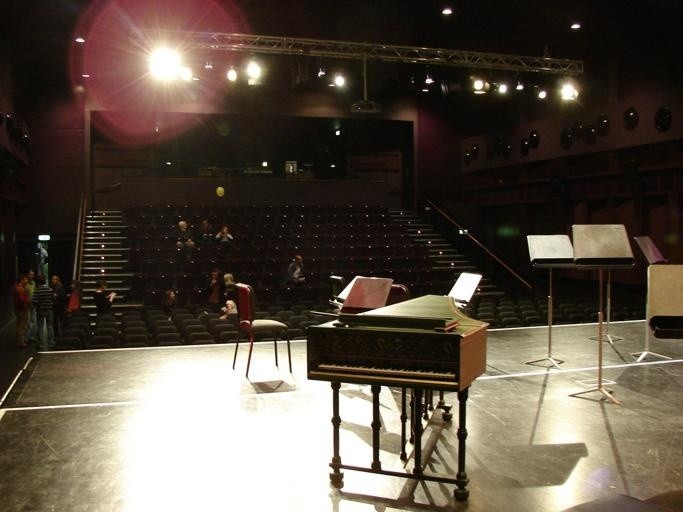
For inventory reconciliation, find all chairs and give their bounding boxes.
[497,295,646,327]
[386,283,409,304]
[231,201,329,296]
[125,203,228,304]
[233,282,292,377]
[468,299,497,328]
[253,297,337,340]
[409,277,481,299]
[330,276,346,296]
[54,306,237,350]
[329,200,444,276]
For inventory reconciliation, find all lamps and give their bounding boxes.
[466,76,490,95]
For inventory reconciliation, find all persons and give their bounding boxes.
[287,255,306,285]
[13,245,82,350]
[192,219,217,245]
[215,224,235,243]
[169,220,195,249]
[93,277,116,318]
[199,268,225,310]
[159,289,177,315]
[223,272,234,303]
[218,300,238,320]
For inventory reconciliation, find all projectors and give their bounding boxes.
[350,101,384,114]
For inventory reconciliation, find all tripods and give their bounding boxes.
[568,267,619,405]
[589,269,623,345]
[525,269,564,369]
[630,266,672,362]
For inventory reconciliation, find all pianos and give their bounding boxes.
[306,295,490,501]
